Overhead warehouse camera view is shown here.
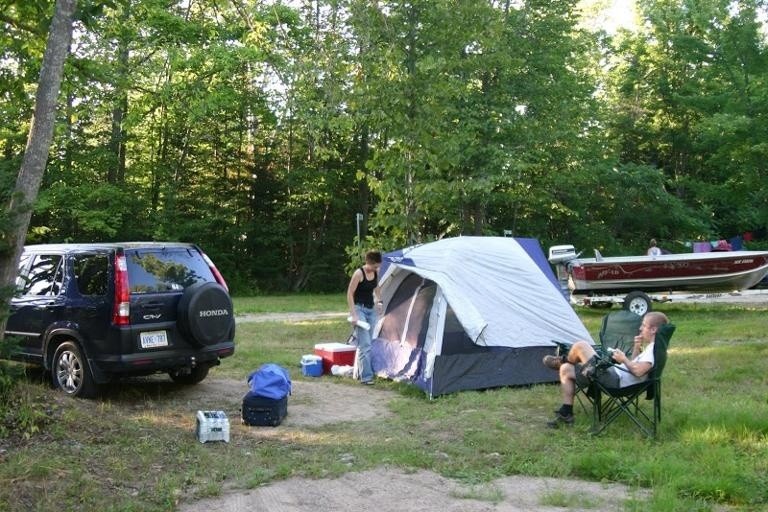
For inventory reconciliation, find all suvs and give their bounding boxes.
[0,240,235,400]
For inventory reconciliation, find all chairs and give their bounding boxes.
[591,323,675,439]
[572,308,642,422]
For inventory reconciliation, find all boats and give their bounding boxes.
[549,244,768,294]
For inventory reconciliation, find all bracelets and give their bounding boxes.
[377,299,383,305]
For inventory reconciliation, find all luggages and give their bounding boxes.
[242,392,288,427]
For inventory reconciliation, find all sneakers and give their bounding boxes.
[547,411,574,427]
[542,355,566,370]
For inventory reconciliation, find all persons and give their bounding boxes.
[543,312,668,428]
[346,249,383,385]
[647,238,662,255]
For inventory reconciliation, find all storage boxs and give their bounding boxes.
[315,342,357,374]
[300,354,324,377]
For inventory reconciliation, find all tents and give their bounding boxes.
[346,236,596,400]
